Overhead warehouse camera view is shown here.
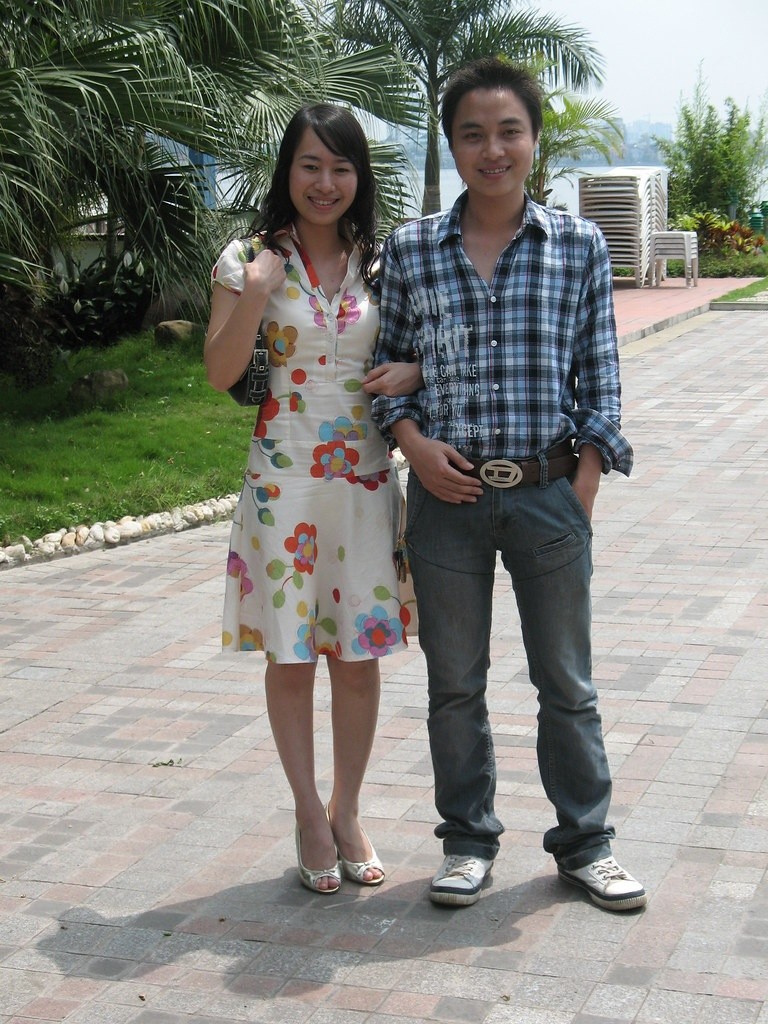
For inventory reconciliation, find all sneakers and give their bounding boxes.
[428,855,493,905]
[557,854,647,910]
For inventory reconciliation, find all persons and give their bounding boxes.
[367,56,648,912]
[205,103,425,895]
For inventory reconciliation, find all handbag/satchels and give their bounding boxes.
[226,238,270,407]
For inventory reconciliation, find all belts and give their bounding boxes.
[449,436,579,488]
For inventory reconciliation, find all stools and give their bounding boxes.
[649,232,698,290]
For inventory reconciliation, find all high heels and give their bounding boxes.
[296,818,341,894]
[326,801,385,886]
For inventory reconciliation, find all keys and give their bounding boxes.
[394,551,400,571]
